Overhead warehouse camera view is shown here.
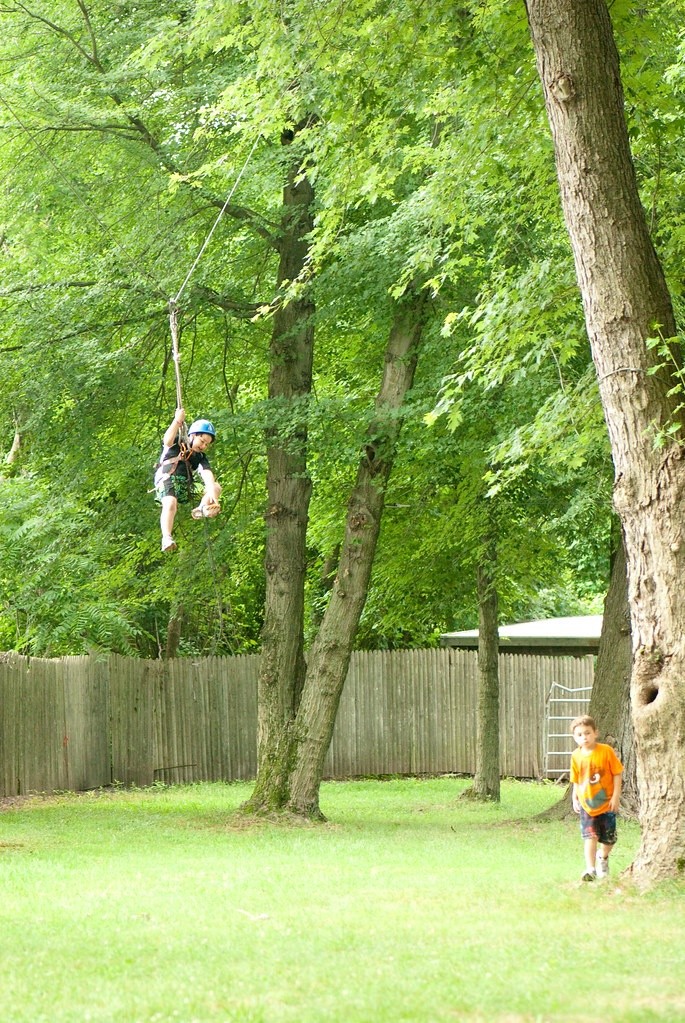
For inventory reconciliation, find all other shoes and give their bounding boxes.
[161,537,177,551]
[190,504,221,520]
[595,848,609,878]
[581,867,596,882]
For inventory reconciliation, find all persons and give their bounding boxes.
[568,715,623,881]
[154,409,222,552]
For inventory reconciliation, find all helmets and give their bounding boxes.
[187,419,215,437]
